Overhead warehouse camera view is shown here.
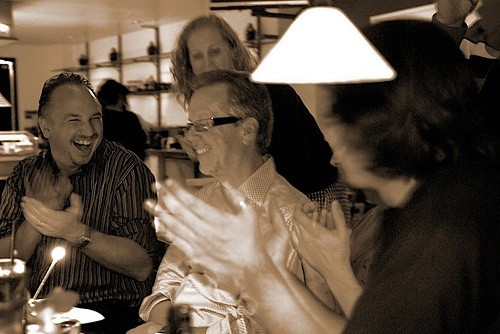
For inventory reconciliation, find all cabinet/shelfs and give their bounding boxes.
[54,25,262,181]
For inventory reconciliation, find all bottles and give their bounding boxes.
[148,41,156,55]
[247,24,255,41]
[110,48,118,61]
[147,76,156,90]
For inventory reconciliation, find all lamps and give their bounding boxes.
[33,246,66,300]
[247,0,400,88]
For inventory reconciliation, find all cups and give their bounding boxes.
[0,257,27,334]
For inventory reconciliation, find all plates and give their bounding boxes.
[27,298,106,330]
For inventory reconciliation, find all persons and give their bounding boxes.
[171,13,341,194]
[290,153,386,319]
[117,69,310,334]
[147,13,500,334]
[96,79,149,162]
[0,72,160,334]
[433,0,500,60]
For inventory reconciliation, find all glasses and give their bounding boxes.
[188,115,250,133]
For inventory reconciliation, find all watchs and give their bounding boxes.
[77,226,91,249]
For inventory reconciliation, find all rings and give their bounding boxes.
[38,222,41,226]
